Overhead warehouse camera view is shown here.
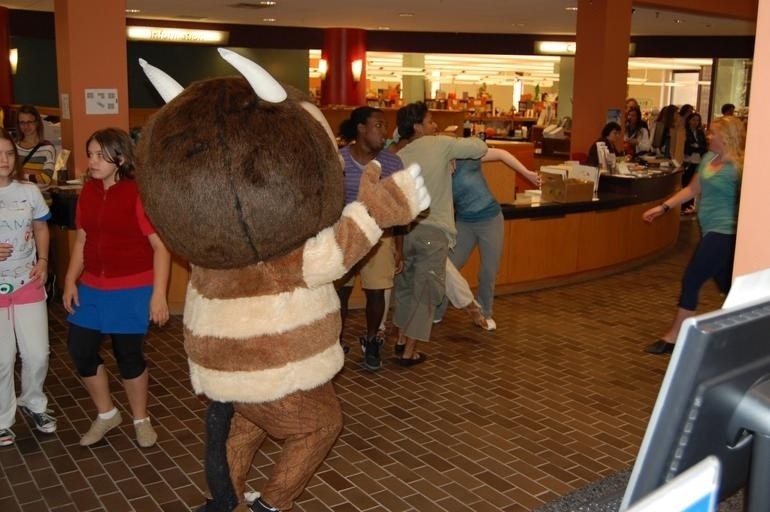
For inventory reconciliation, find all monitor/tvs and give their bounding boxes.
[652,121,671,159]
[619,297,770,512]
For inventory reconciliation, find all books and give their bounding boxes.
[596,140,650,177]
[536,103,557,127]
[538,157,599,182]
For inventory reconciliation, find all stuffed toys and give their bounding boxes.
[139,45,430,510]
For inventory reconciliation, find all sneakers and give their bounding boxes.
[21,406,58,433]
[481,318,497,331]
[359,334,383,370]
[249,498,282,512]
[45,270,57,305]
[0,428,17,446]
[79,409,123,446]
[133,418,158,448]
[395,345,405,353]
[399,352,426,366]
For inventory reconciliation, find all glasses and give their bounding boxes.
[17,121,37,126]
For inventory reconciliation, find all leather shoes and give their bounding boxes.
[643,339,675,355]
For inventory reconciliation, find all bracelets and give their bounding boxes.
[37,255,48,262]
[661,201,669,214]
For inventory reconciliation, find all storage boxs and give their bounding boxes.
[540,171,594,204]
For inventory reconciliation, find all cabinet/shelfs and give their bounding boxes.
[464,115,539,142]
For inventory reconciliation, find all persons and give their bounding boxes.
[65,129,170,447]
[588,98,738,212]
[644,116,751,355]
[360,102,541,363]
[338,106,404,369]
[0,132,57,447]
[13,105,51,205]
